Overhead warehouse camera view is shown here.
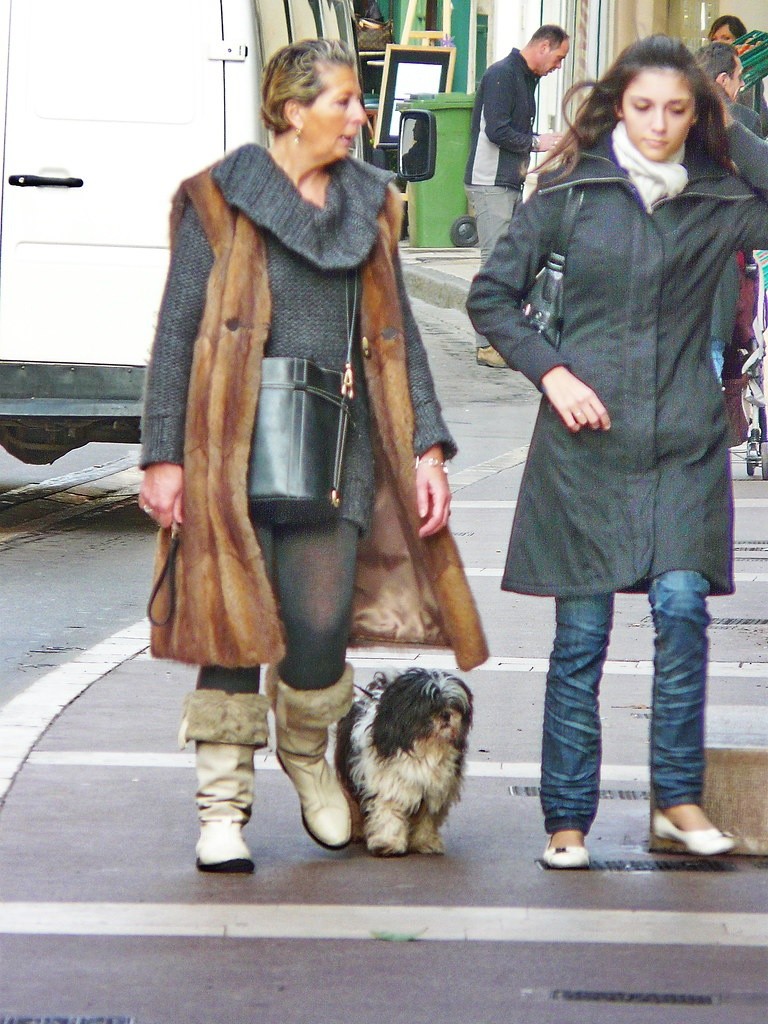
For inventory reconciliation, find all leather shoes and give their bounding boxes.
[650,808,736,856]
[542,831,587,866]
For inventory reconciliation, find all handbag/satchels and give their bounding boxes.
[246,355,353,520]
[520,183,585,351]
[354,14,395,52]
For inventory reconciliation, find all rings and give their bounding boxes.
[144,506,153,513]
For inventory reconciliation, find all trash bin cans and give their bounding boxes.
[395,92,478,248]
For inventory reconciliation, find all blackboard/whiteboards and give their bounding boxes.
[373,43,457,146]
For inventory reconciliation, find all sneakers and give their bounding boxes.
[476,346,508,368]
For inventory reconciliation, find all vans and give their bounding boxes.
[2,0,438,467]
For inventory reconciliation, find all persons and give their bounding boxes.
[708,15,768,138]
[692,42,764,389]
[138,38,493,873]
[466,26,566,369]
[463,31,768,869]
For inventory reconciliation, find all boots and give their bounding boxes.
[266,657,355,849]
[179,689,269,873]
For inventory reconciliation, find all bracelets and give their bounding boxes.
[415,456,448,475]
[533,136,540,151]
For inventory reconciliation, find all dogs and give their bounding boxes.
[334,668,474,857]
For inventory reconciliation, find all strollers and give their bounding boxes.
[730,260,768,481]
